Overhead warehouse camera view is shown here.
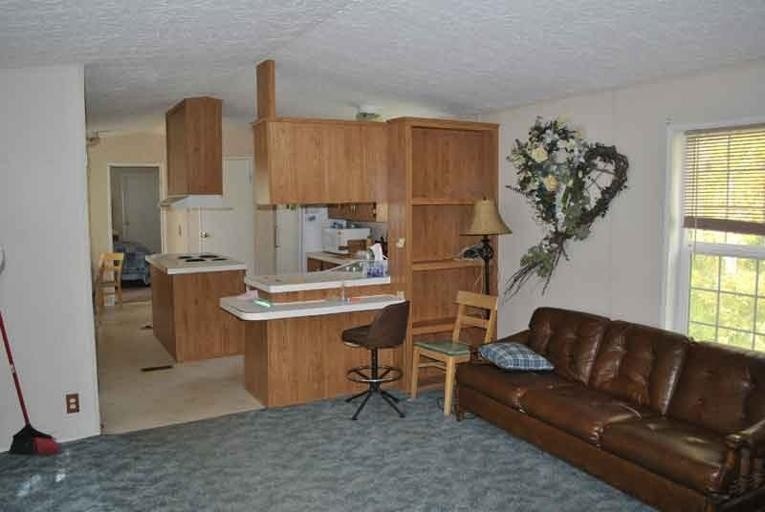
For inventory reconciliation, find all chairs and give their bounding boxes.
[95,251,126,311]
[340,290,501,422]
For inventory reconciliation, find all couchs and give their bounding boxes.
[453,304,765,511]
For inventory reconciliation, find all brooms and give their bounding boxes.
[0,309,61,454]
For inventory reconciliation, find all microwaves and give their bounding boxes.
[322,225,373,256]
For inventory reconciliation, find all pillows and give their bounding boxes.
[476,340,558,373]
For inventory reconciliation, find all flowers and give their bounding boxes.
[506,110,587,231]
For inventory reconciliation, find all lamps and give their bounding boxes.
[459,194,513,319]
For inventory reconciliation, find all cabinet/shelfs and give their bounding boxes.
[386,116,501,393]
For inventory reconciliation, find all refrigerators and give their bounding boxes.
[274,203,332,275]
